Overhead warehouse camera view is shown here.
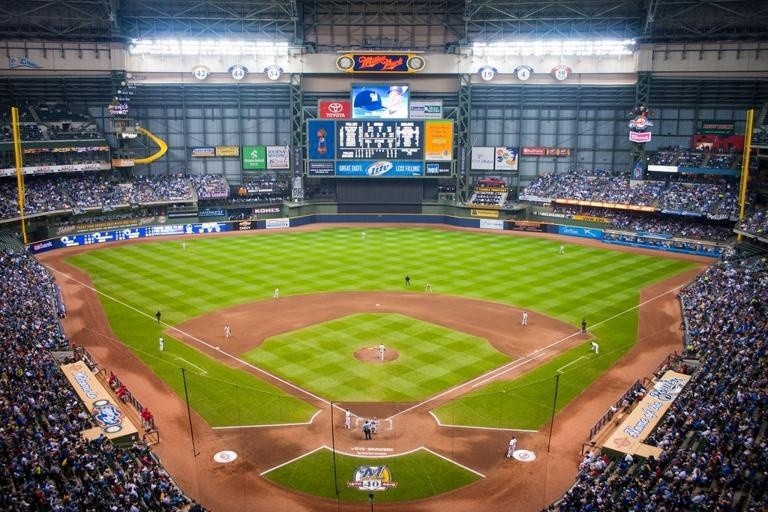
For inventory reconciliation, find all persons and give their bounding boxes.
[650,142,744,171]
[1,171,292,223]
[344,408,351,429]
[0,249,205,512]
[224,325,230,338]
[406,276,410,286]
[425,283,432,295]
[363,417,379,441]
[542,245,768,512]
[273,289,280,298]
[381,86,408,118]
[378,344,386,359]
[518,170,768,246]
[0,103,111,170]
[522,312,528,327]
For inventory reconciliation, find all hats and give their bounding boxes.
[354,90,389,111]
[388,86,403,96]
